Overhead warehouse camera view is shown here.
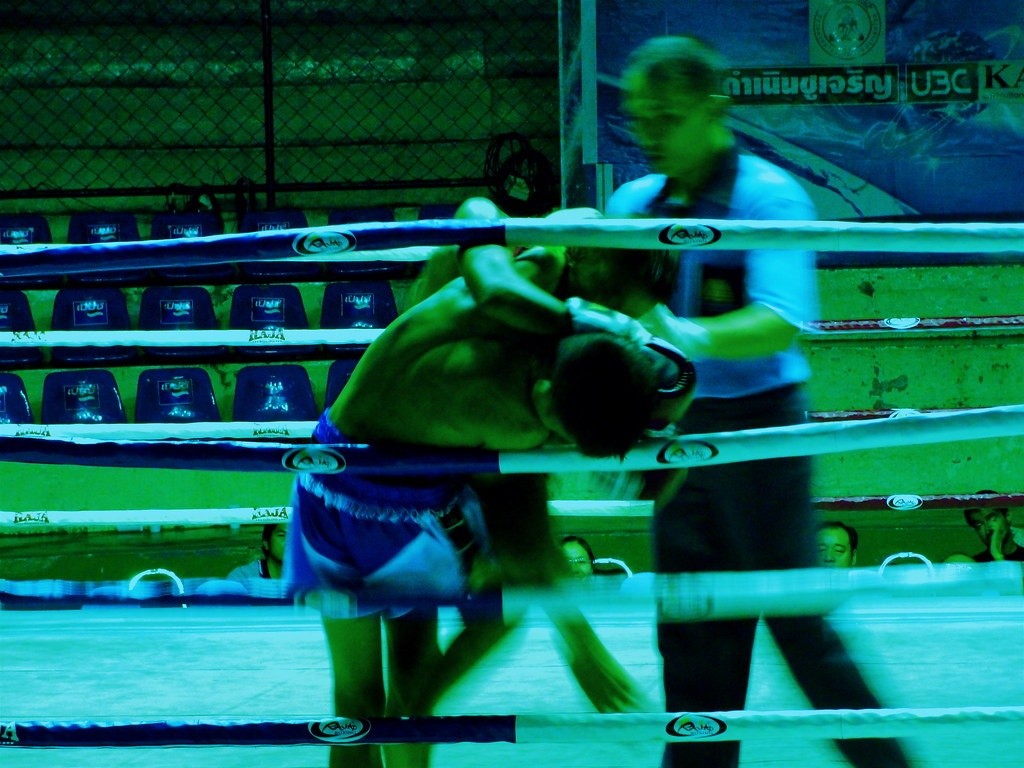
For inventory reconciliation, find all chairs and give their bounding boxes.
[0,204,458,461]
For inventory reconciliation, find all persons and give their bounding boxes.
[815,521,858,571]
[557,535,596,589]
[281,43,909,768]
[226,523,289,591]
[964,490,1024,562]
